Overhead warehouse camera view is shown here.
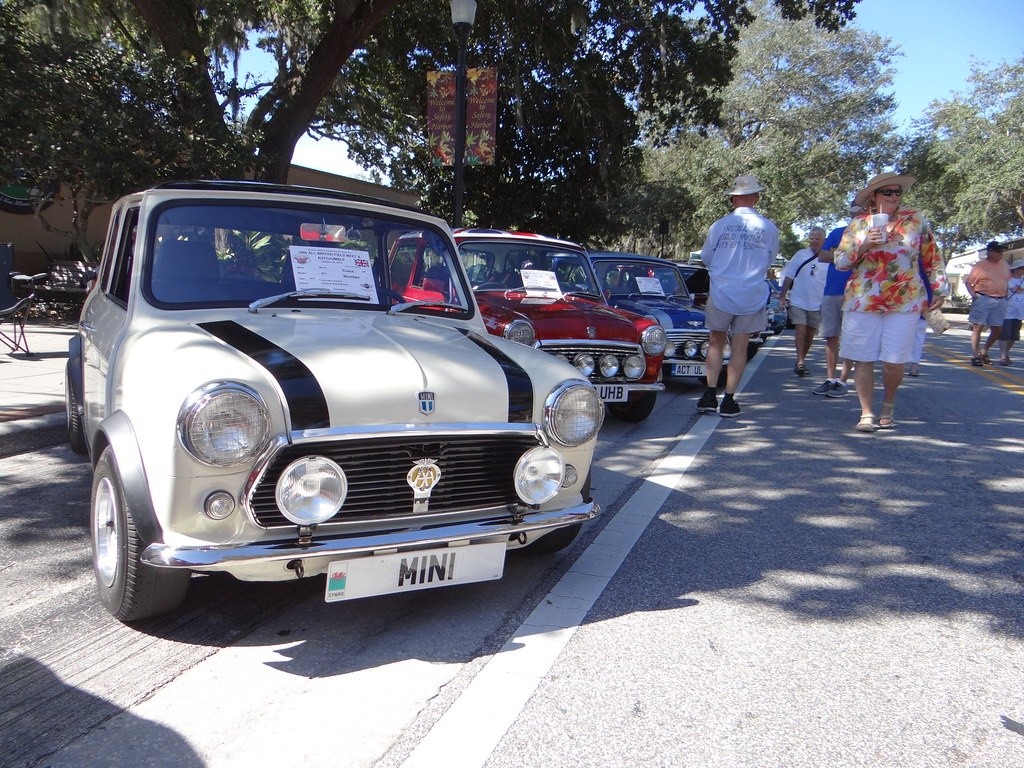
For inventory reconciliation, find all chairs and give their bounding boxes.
[425,264,450,292]
[0,243,47,357]
[152,238,224,304]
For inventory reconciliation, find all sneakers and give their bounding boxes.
[813,380,834,394]
[827,382,848,397]
[971,357,983,366]
[720,400,741,417]
[978,351,993,364]
[695,397,718,411]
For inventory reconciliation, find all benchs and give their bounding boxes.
[21,262,100,324]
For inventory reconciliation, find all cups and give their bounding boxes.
[870,214,888,244]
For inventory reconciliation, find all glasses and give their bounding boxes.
[876,189,903,196]
[989,249,1004,253]
[810,263,815,275]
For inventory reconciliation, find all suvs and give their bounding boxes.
[373,222,670,425]
[62,176,608,626]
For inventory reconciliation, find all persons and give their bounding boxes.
[909,254,934,375]
[696,176,780,416]
[780,226,829,376]
[834,171,950,432]
[996,260,1024,365]
[964,241,1012,365]
[811,200,871,397]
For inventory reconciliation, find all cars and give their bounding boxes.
[519,247,796,389]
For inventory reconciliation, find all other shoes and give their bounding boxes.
[1007,356,1015,366]
[794,364,810,373]
[999,358,1007,366]
[796,364,805,375]
[910,366,919,375]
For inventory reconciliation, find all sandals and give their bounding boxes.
[878,401,894,427]
[857,414,875,432]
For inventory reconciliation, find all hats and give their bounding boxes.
[729,175,764,195]
[854,172,917,207]
[986,241,1008,249]
[1009,260,1024,269]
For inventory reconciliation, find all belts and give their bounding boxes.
[975,291,1004,299]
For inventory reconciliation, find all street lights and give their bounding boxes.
[442,0,480,229]
